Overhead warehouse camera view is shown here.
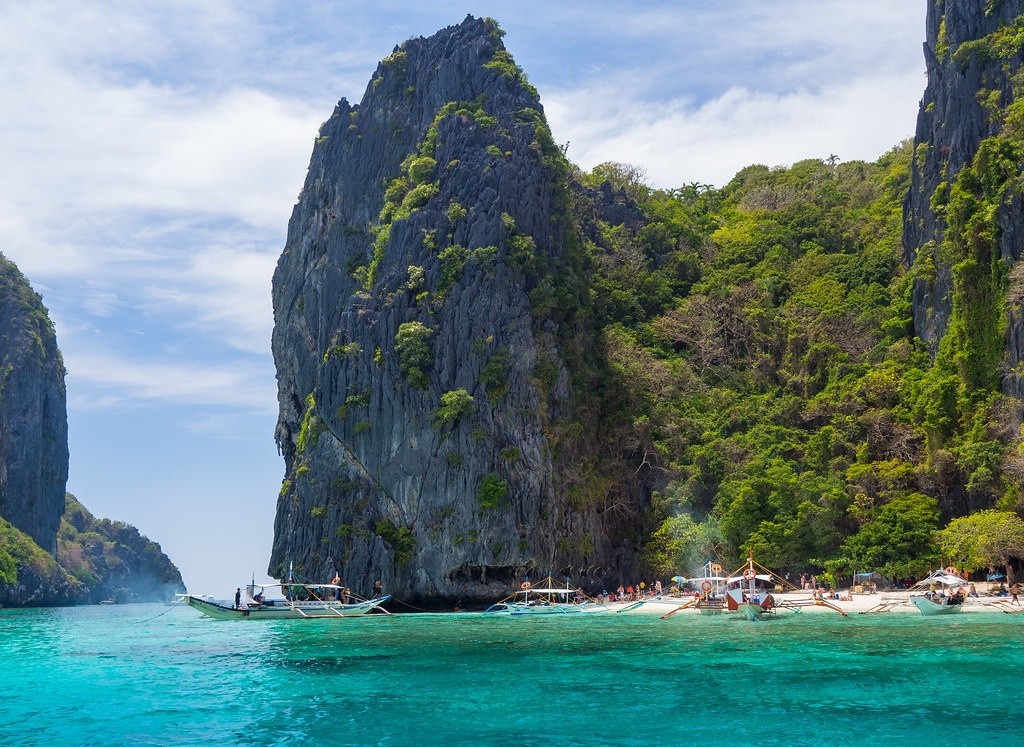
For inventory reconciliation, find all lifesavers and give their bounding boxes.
[522,581,530,589]
[743,567,757,580]
[712,564,722,573]
[702,581,712,591]
[947,567,957,575]
[332,577,340,584]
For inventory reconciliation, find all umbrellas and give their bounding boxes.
[671,576,686,583]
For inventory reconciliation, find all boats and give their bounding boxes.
[504,570,582,615]
[165,593,225,606]
[726,548,775,617]
[908,555,967,616]
[686,561,727,609]
[184,560,392,623]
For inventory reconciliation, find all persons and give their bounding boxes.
[785,572,790,583]
[1011,583,1021,606]
[609,591,616,602]
[927,578,998,605]
[847,591,853,601]
[760,582,764,591]
[812,590,817,604]
[811,574,816,590]
[235,588,240,610]
[598,592,604,604]
[307,583,344,603]
[627,585,633,602]
[342,587,350,603]
[617,585,625,604]
[800,572,806,589]
[635,579,661,599]
[372,581,382,600]
[253,592,262,602]
[830,589,835,599]
[764,592,775,606]
[818,587,823,600]
